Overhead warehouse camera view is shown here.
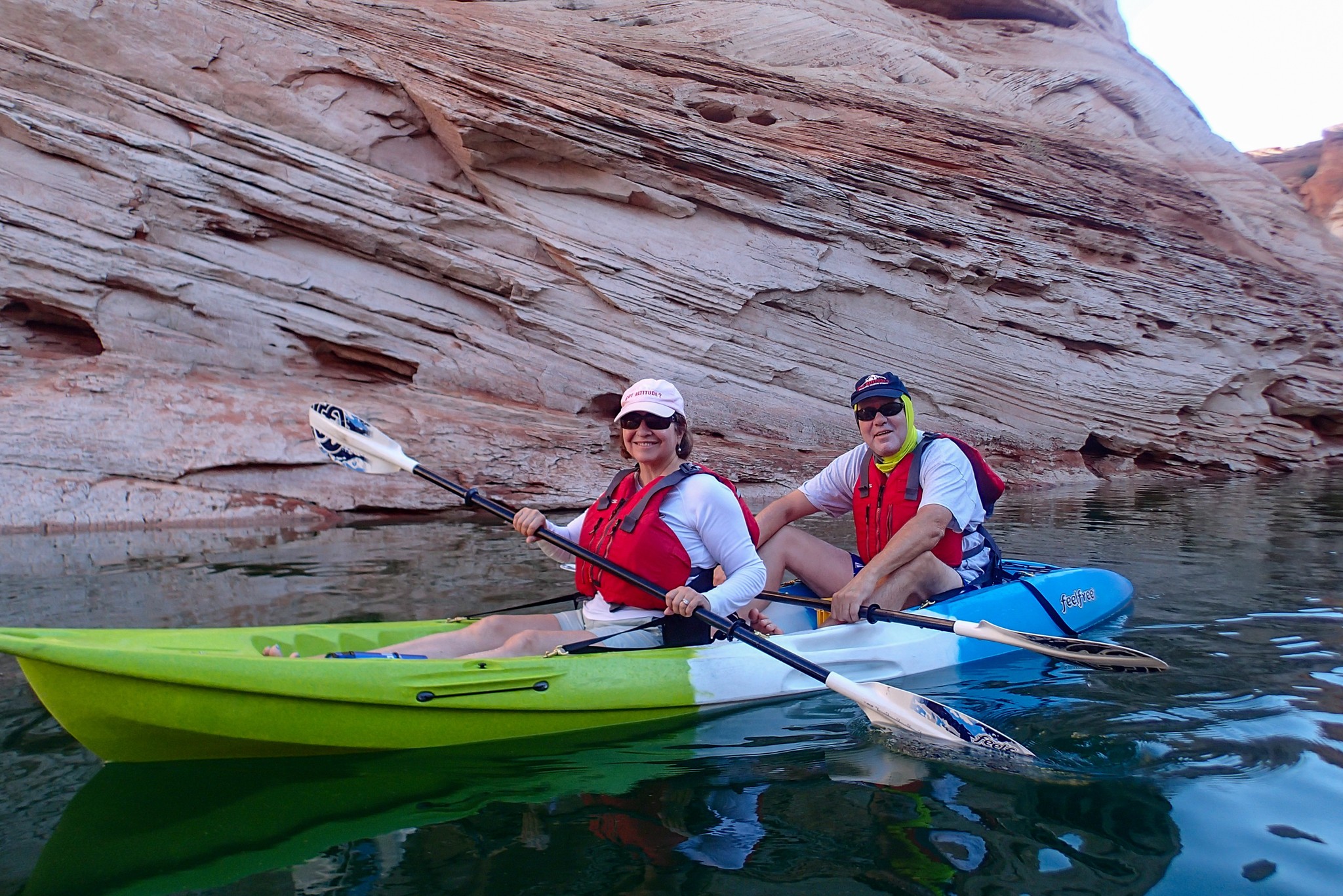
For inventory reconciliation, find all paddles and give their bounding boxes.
[560,563,1168,673]
[309,402,1036,755]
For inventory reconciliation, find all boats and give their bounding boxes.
[11,607,1133,896]
[0,558,1138,760]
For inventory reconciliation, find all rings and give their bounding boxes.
[682,598,689,605]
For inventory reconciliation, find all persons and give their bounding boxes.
[262,378,766,659]
[713,372,990,635]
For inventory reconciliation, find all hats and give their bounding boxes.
[613,378,687,424]
[851,371,911,407]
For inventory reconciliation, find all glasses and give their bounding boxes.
[857,402,904,421]
[620,412,676,430]
